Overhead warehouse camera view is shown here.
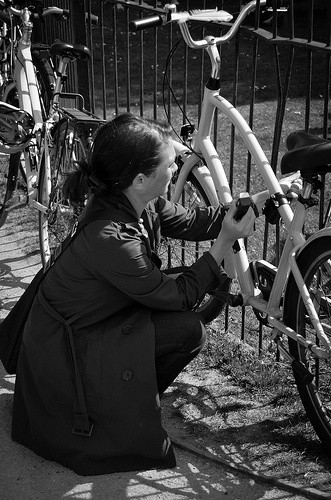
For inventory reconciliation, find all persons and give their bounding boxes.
[2,112,302,477]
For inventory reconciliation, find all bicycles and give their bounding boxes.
[131,1,331,464]
[0,0,108,265]
